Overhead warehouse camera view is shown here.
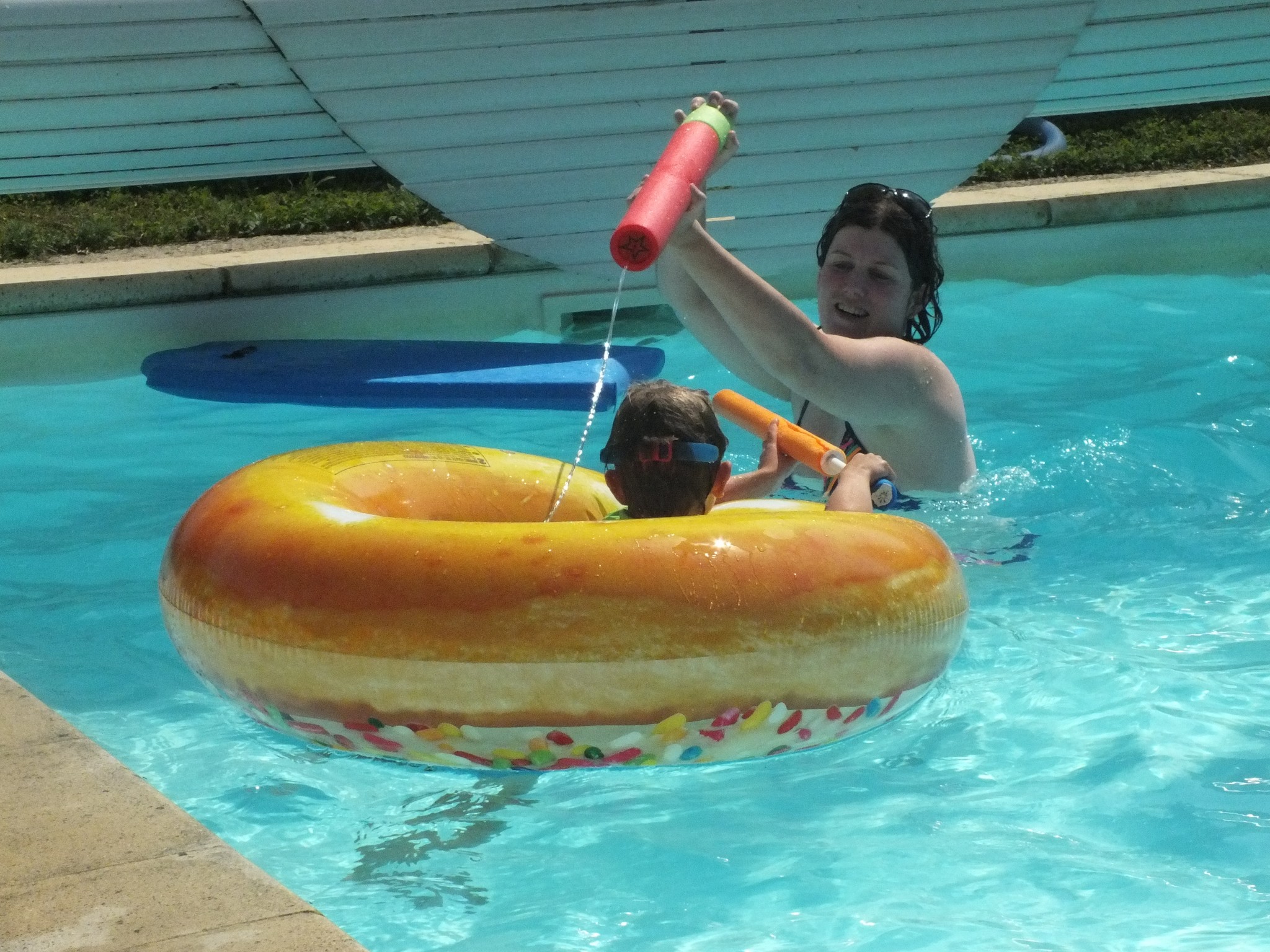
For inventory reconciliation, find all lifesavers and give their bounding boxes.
[154,438,971,771]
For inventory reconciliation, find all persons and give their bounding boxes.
[624,90,979,493]
[598,381,897,522]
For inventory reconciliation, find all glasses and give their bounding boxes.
[842,182,933,227]
